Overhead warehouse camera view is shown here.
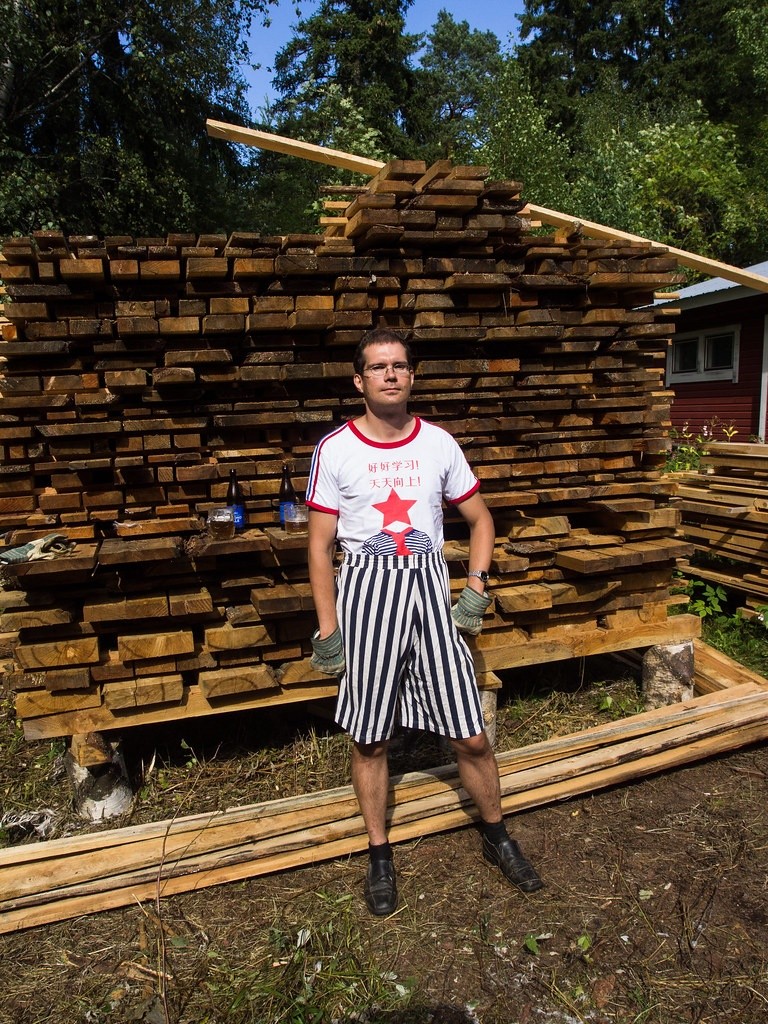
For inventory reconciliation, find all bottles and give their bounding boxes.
[278,465,297,530]
[226,469,245,534]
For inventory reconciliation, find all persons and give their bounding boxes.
[307,332,547,916]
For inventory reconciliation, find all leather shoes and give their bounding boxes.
[364,858,397,915]
[482,833,543,890]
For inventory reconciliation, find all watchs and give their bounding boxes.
[467,570,489,583]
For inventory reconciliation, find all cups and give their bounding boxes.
[283,503,309,535]
[208,507,234,540]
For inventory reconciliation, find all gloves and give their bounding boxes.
[311,626,345,675]
[450,585,492,637]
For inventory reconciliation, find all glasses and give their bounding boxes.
[361,364,411,376]
